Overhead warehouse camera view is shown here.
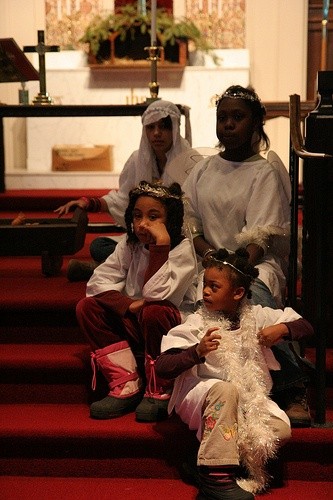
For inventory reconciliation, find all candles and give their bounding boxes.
[151,0,157,46]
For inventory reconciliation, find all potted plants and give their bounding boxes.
[77,6,224,88]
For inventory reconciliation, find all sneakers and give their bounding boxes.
[286,397,312,426]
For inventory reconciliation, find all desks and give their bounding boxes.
[0,104,192,193]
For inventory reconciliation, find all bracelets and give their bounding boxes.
[203,249,217,257]
[81,197,102,212]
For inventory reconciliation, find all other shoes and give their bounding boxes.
[67,258,100,280]
[89,395,139,417]
[138,397,170,422]
[202,481,256,500]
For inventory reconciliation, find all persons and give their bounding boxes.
[76,180,201,422]
[152,245,315,500]
[55,100,202,283]
[181,85,316,426]
